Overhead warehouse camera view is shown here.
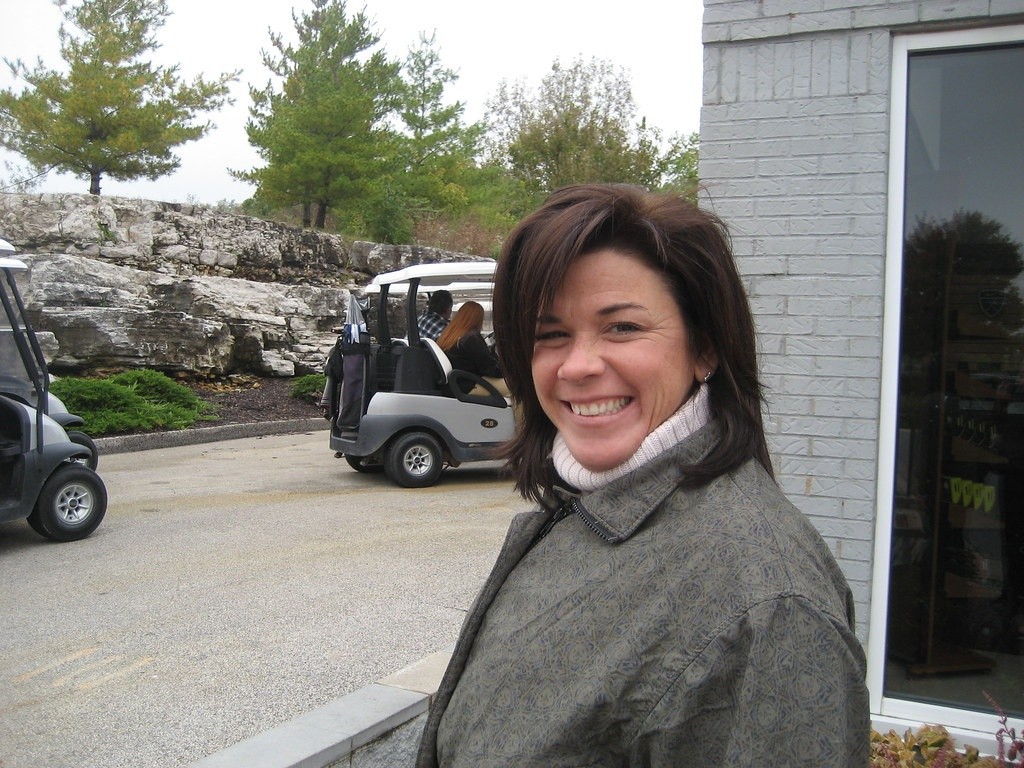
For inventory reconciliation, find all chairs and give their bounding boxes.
[390,337,508,408]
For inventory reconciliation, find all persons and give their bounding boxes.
[414,184,872,767]
[406,287,512,401]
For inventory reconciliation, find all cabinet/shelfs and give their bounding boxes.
[926,238,1023,666]
